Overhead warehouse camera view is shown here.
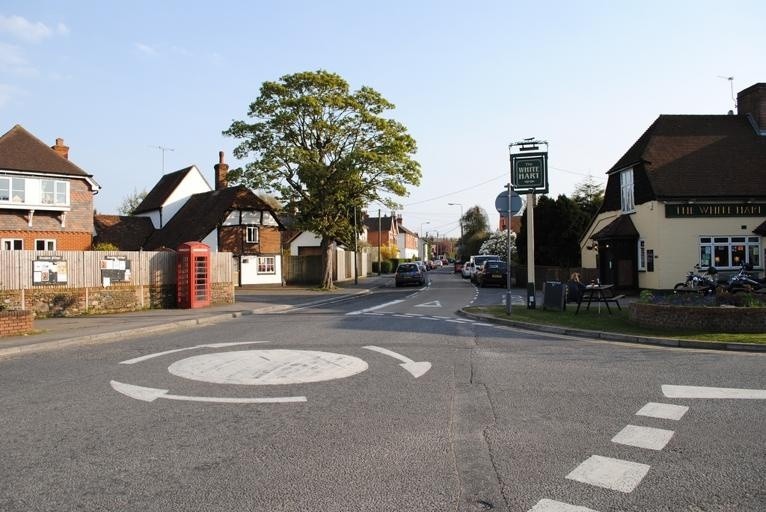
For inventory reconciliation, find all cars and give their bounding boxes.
[396,258,448,285]
[454,254,507,287]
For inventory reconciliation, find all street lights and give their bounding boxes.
[159,146,174,175]
[421,222,430,238]
[448,202,464,254]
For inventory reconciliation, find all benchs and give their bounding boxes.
[577,295,625,302]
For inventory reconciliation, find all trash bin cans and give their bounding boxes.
[542,281,568,312]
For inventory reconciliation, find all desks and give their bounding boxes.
[576,284,621,314]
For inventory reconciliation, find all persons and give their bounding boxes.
[565,272,585,303]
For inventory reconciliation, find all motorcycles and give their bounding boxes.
[672,262,764,297]
